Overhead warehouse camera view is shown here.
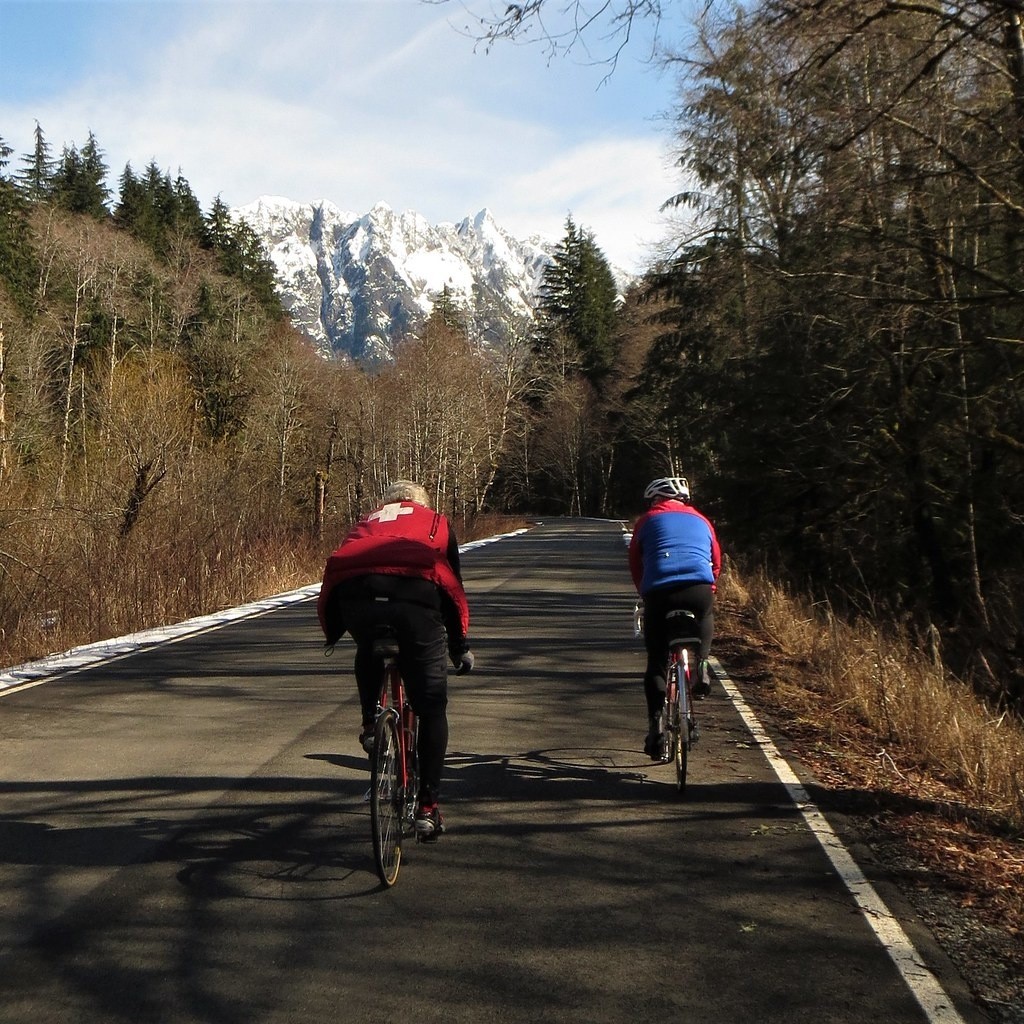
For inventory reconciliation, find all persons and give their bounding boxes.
[629,475,723,759]
[316,480,474,836]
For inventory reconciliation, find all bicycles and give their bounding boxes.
[359,633,462,887]
[633,604,719,794]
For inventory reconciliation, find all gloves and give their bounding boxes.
[449,650,475,676]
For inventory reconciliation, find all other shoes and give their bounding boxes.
[645,733,664,756]
[693,654,712,695]
[416,802,447,834]
[359,726,386,753]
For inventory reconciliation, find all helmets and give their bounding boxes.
[643,477,690,504]
